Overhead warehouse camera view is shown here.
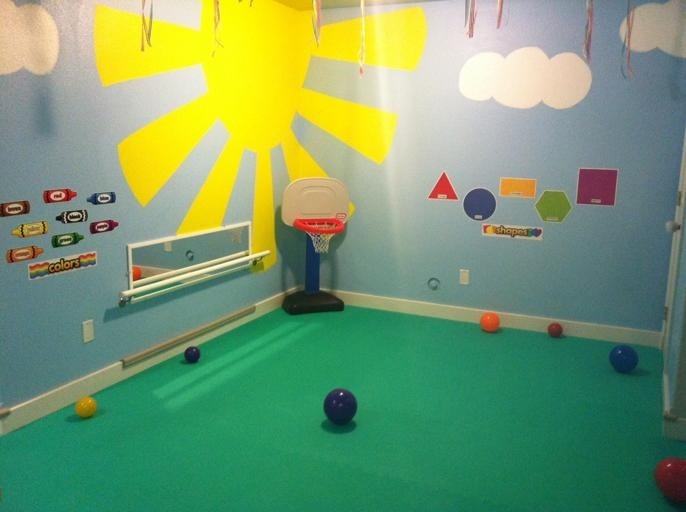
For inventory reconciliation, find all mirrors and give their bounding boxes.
[127,220,252,309]
